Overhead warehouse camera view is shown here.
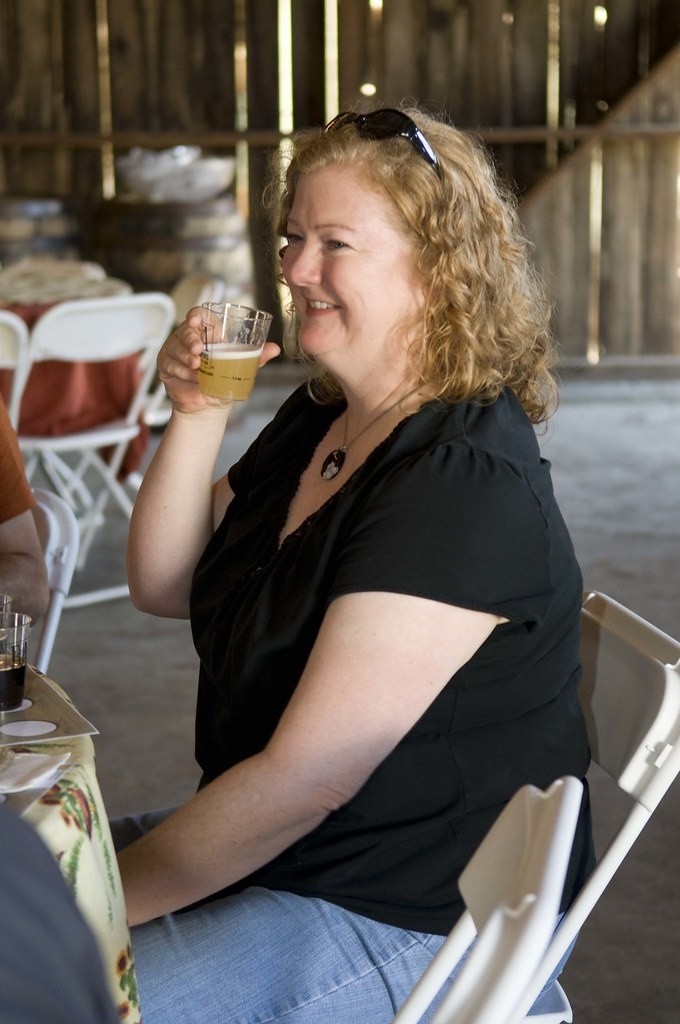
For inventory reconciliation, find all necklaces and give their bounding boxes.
[320,381,427,481]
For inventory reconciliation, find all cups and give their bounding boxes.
[0,591,14,612]
[192,301,274,407]
[0,612,32,712]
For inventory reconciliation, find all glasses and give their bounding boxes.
[323,110,440,172]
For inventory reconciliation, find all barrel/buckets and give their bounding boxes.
[98,194,255,297]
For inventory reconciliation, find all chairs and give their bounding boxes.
[390,591,680,1024]
[0,274,224,677]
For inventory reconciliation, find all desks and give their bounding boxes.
[0,656,142,1024]
[0,268,137,556]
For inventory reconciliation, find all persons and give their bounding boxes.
[111,98,600,1024]
[0,397,53,636]
